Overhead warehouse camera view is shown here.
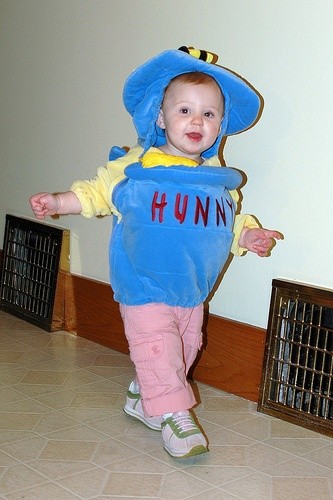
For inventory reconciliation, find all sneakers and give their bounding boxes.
[160,409,209,459]
[123,381,166,432]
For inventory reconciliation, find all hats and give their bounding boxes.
[120,46,264,157]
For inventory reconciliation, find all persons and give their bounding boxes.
[29,46,285,461]
